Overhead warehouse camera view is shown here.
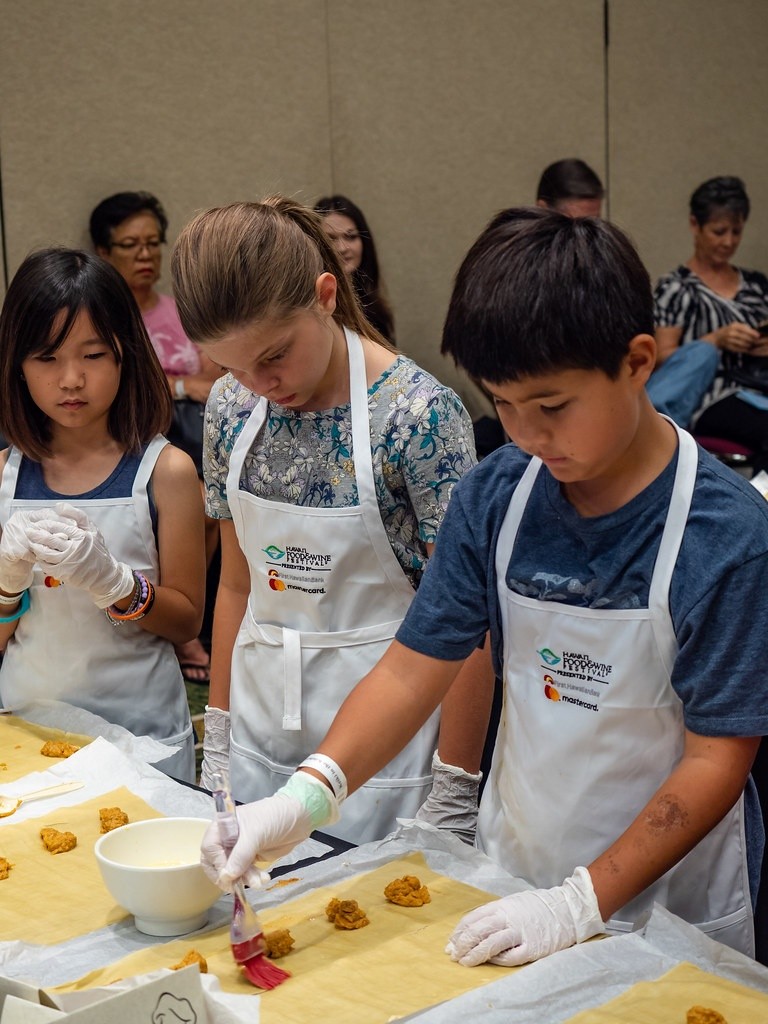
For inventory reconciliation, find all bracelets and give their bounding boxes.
[0,589,30,623]
[102,568,155,628]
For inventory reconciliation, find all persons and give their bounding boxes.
[0,248,204,787]
[650,176,768,429]
[536,159,603,222]
[169,193,477,848]
[203,206,768,970]
[88,190,228,686]
[309,195,396,350]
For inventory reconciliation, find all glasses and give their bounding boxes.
[107,233,168,254]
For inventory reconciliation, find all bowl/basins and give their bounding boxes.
[93,814,232,937]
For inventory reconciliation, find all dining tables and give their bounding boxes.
[0,768,766,1024]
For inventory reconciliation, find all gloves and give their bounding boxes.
[23,500,134,611]
[198,703,231,793]
[445,866,606,968]
[415,749,484,848]
[1,508,77,595]
[199,770,342,895]
